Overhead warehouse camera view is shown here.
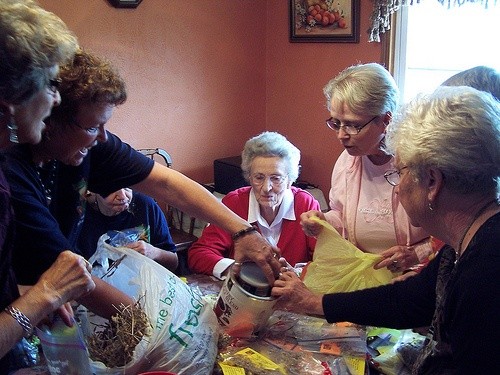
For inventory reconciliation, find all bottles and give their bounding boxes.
[213,262,279,338]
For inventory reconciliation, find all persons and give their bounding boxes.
[299,62,445,274]
[270,84,500,375]
[186,132,322,283]
[442,65,500,100]
[68,187,179,272]
[14,48,286,286]
[1,0,146,375]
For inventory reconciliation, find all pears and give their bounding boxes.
[307,0,345,28]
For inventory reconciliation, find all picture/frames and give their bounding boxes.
[289,0,360,43]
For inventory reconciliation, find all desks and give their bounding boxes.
[6,272,368,375]
[166,184,328,238]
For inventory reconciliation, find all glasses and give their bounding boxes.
[325,116,377,135]
[384,165,408,186]
[45,78,63,93]
[248,171,289,186]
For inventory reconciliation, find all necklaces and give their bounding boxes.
[35,160,56,206]
[454,199,498,265]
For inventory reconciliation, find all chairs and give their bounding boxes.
[136,149,215,252]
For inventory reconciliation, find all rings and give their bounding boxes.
[234,262,244,266]
[393,259,397,264]
[272,252,277,257]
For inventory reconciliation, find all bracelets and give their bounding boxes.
[3,305,35,338]
[231,225,258,240]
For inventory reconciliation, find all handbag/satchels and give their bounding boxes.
[80,232,219,375]
